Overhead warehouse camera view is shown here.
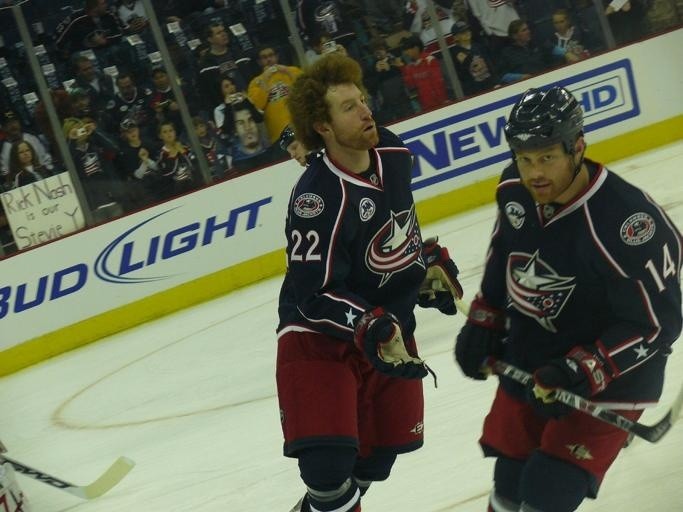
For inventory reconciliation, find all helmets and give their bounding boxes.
[280,123,297,151]
[503,85,583,149]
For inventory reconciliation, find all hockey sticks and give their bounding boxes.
[0,454,135,500]
[497,358,683,444]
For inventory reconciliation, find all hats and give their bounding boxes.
[451,21,469,34]
[120,117,137,129]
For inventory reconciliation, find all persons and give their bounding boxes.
[277,54,464,512]
[455,87,683,512]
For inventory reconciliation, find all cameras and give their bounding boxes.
[229,93,242,101]
[268,66,278,73]
[76,127,87,136]
[323,41,337,53]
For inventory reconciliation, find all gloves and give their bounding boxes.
[528,338,619,418]
[456,293,505,380]
[416,236,462,315]
[354,308,428,379]
